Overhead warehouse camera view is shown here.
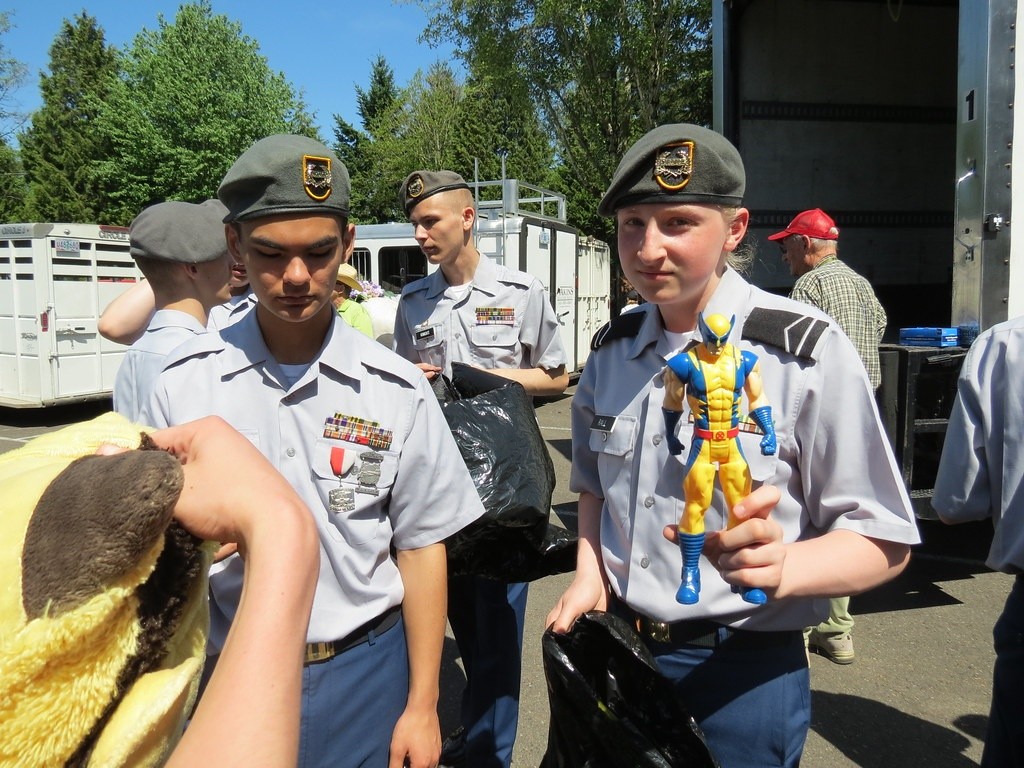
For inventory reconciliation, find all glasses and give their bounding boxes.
[778,241,787,254]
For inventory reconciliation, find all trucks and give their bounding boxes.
[0,222,146,410]
[346,155,611,379]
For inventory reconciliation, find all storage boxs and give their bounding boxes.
[899,326,960,347]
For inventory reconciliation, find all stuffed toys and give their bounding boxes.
[0,412,220,768]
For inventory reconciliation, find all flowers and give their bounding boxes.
[350,279,397,300]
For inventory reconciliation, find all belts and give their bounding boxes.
[199,606,402,693]
[606,595,795,649]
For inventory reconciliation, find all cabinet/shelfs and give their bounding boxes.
[876,343,969,521]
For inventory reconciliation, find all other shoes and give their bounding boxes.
[810,633,855,665]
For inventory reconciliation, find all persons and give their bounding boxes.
[392,171,570,768]
[329,263,374,341]
[97,258,249,346]
[620,290,640,316]
[546,122,922,768]
[662,311,777,605]
[147,416,320,768]
[136,136,487,768]
[767,208,888,664]
[113,200,237,427]
[931,316,1024,768]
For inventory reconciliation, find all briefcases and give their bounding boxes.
[899,326,961,347]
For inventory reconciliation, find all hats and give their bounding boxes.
[217,135,352,225]
[768,209,840,241]
[129,198,230,263]
[597,125,745,218]
[398,170,469,220]
[337,263,363,293]
